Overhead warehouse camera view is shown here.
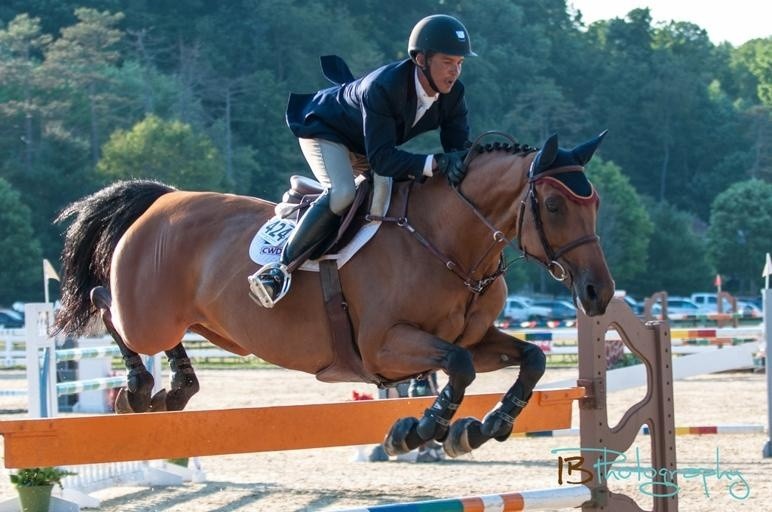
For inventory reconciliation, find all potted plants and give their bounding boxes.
[10,462,78,511]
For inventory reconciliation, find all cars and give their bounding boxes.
[624,292,762,315]
[504,297,576,322]
[0,308,25,329]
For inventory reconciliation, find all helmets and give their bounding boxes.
[407,14,478,64]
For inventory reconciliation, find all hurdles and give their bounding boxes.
[0,291,677,512]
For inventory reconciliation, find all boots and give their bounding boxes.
[256,185,343,303]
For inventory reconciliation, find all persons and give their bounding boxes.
[247,14,479,311]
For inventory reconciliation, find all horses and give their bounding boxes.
[46,123,618,467]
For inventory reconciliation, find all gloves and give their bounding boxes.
[438,149,469,184]
